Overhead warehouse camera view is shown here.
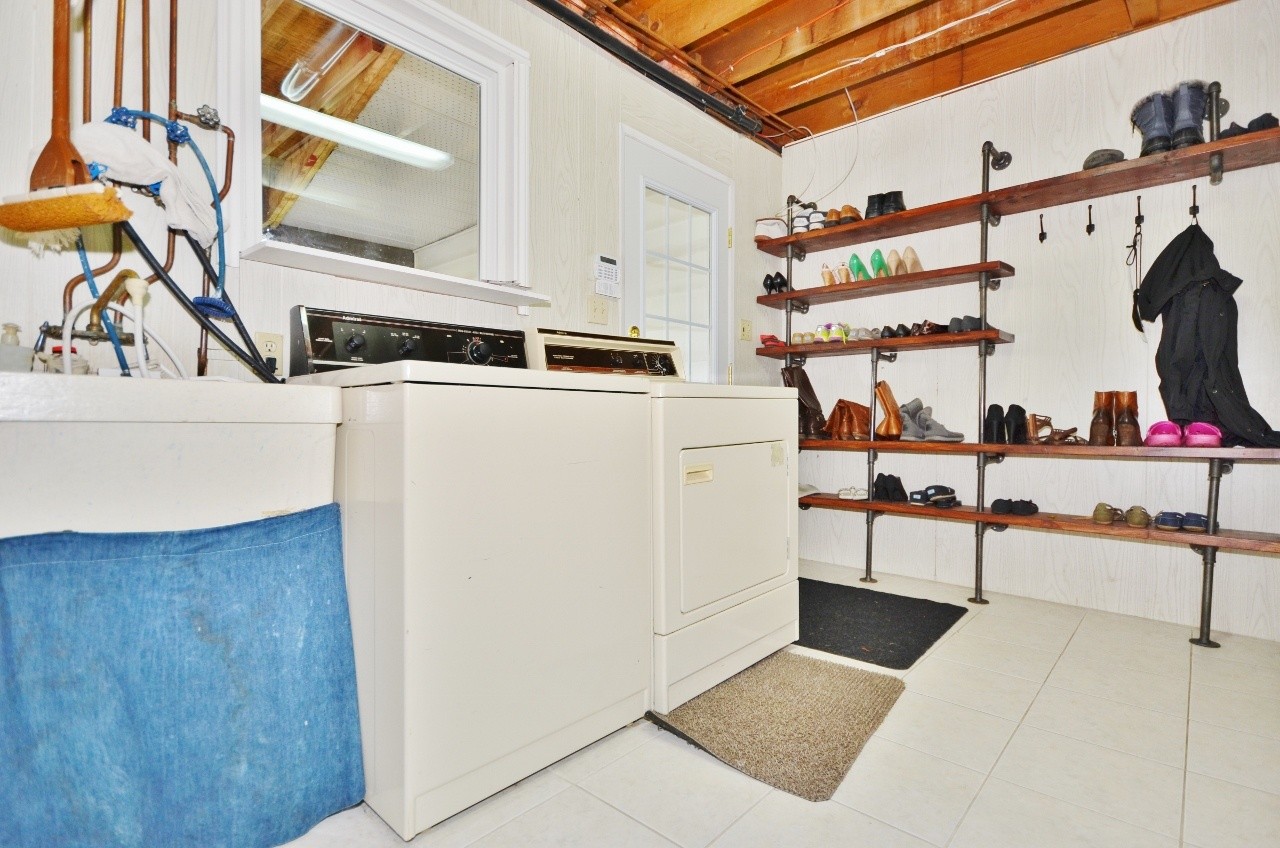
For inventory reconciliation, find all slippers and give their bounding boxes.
[1183,419,1225,450]
[1145,421,1183,450]
[838,485,869,501]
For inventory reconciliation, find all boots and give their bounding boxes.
[1128,90,1170,157]
[873,380,967,442]
[1167,80,1208,147]
[781,366,871,441]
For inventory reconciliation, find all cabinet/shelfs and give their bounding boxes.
[757,84,1280,650]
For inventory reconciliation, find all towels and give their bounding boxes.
[69,122,231,250]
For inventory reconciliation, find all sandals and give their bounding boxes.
[1093,502,1125,525]
[1127,505,1154,529]
[1025,413,1089,446]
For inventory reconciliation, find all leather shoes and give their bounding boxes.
[864,193,885,218]
[883,190,909,215]
[839,204,862,223]
[826,209,840,227]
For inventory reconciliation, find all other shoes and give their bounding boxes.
[791,213,808,235]
[1156,510,1184,533]
[909,485,963,509]
[808,210,826,232]
[990,498,1039,515]
[759,315,999,348]
[1182,512,1220,534]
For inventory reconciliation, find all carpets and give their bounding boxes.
[644,650,904,803]
[795,574,970,671]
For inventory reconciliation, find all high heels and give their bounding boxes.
[874,472,909,502]
[1003,404,1029,445]
[1116,388,1144,445]
[982,403,1005,444]
[1087,390,1114,446]
[821,245,925,287]
[771,271,797,293]
[761,273,774,295]
